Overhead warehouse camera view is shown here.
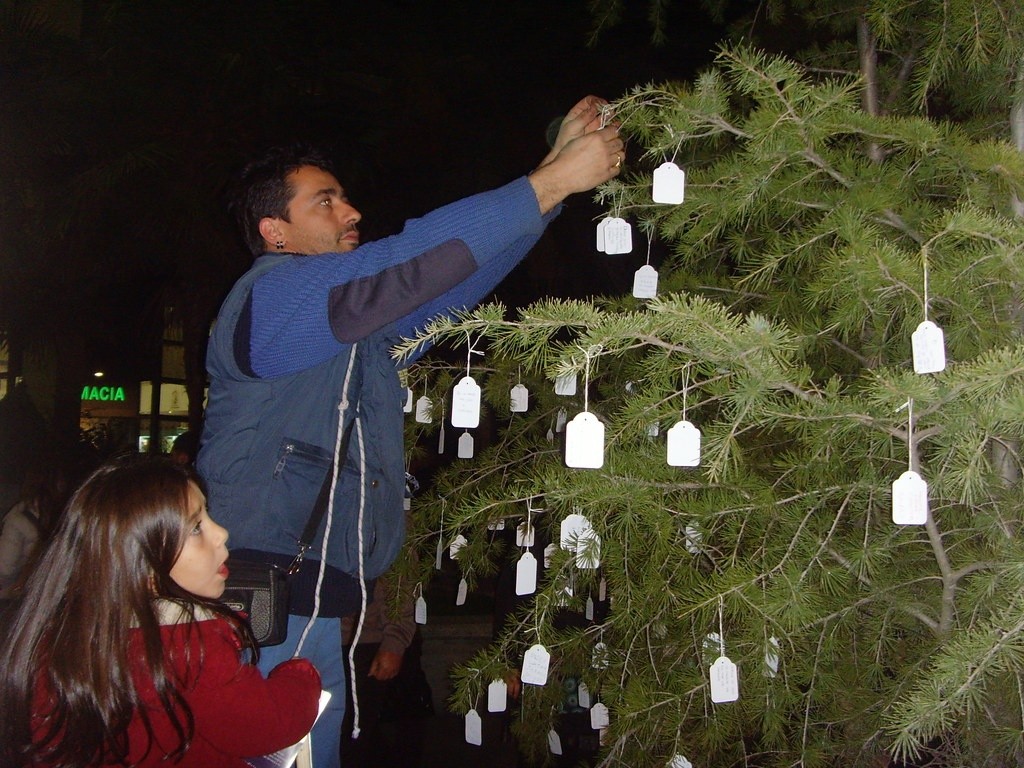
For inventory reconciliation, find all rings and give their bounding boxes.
[614,153,623,168]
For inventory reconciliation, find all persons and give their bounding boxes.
[0,453,322,768]
[196,96,626,768]
[493,528,544,768]
[339,510,423,768]
[0,432,199,604]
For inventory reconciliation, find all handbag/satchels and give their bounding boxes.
[207,560,296,648]
